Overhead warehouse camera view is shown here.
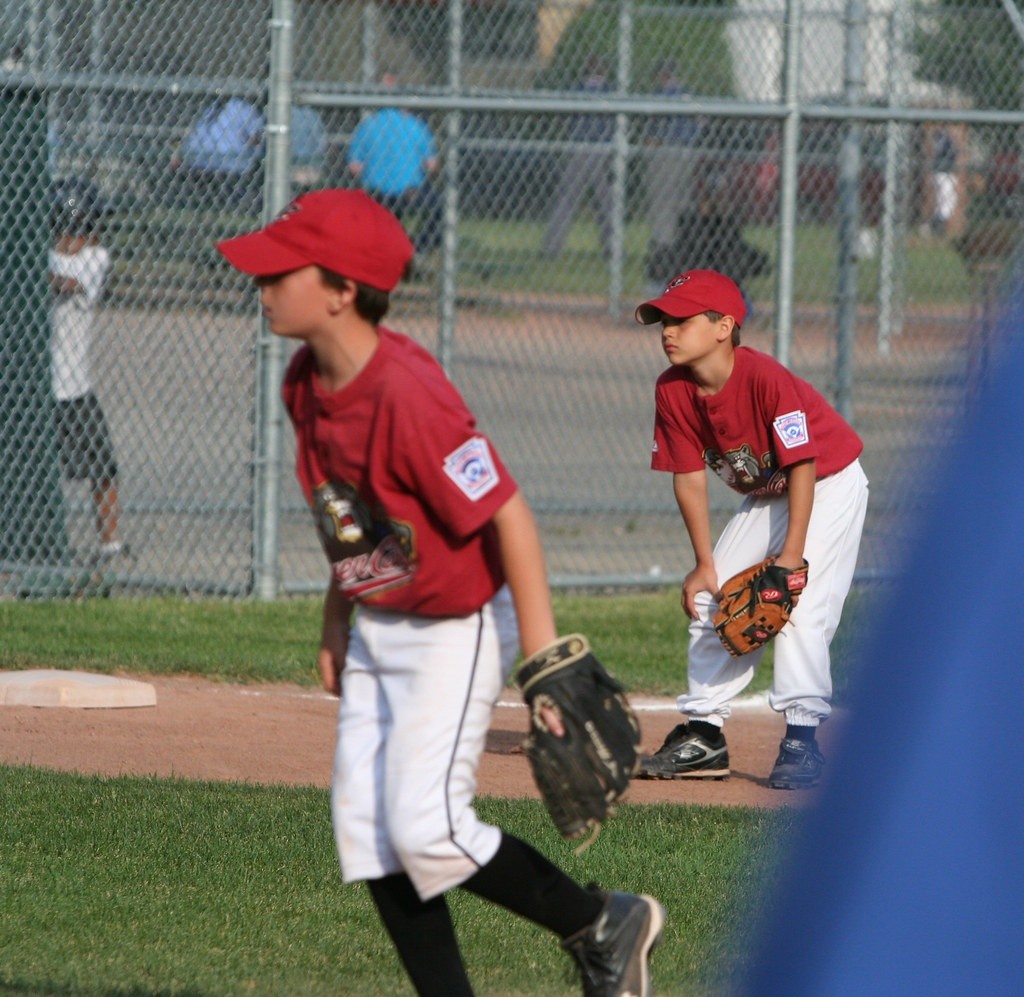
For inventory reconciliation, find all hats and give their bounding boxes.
[634,270,748,328]
[215,189,413,294]
[48,180,101,235]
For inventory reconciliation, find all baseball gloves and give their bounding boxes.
[508,632,643,843]
[712,552,810,661]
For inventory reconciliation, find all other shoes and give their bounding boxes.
[89,544,140,572]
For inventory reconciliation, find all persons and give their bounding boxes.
[634,270,869,791]
[541,53,615,264]
[926,119,964,240]
[46,178,136,564]
[634,58,701,258]
[346,105,440,218]
[215,189,662,997]
[172,95,328,215]
[722,310,1024,997]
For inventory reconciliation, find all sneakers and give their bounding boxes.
[559,881,663,997]
[633,724,730,780]
[768,738,825,789]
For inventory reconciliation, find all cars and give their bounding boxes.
[682,115,882,226]
